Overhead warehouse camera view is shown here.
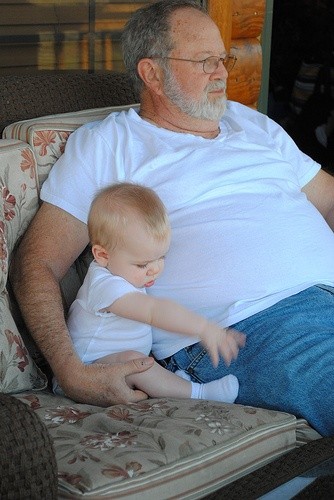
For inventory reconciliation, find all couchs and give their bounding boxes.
[0,67,333,500]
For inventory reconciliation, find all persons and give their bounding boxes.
[7,0,334,442]
[50,180,247,407]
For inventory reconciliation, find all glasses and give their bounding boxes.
[144,54,238,75]
